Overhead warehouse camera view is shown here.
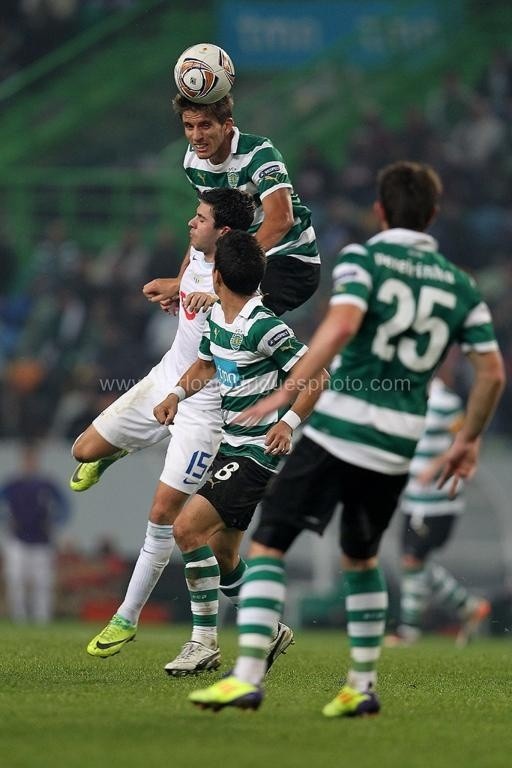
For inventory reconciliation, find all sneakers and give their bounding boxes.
[454,598,492,648]
[321,684,380,719]
[69,450,129,492]
[87,614,137,659]
[384,634,415,648]
[164,621,295,713]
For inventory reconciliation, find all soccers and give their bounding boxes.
[173,43,236,104]
